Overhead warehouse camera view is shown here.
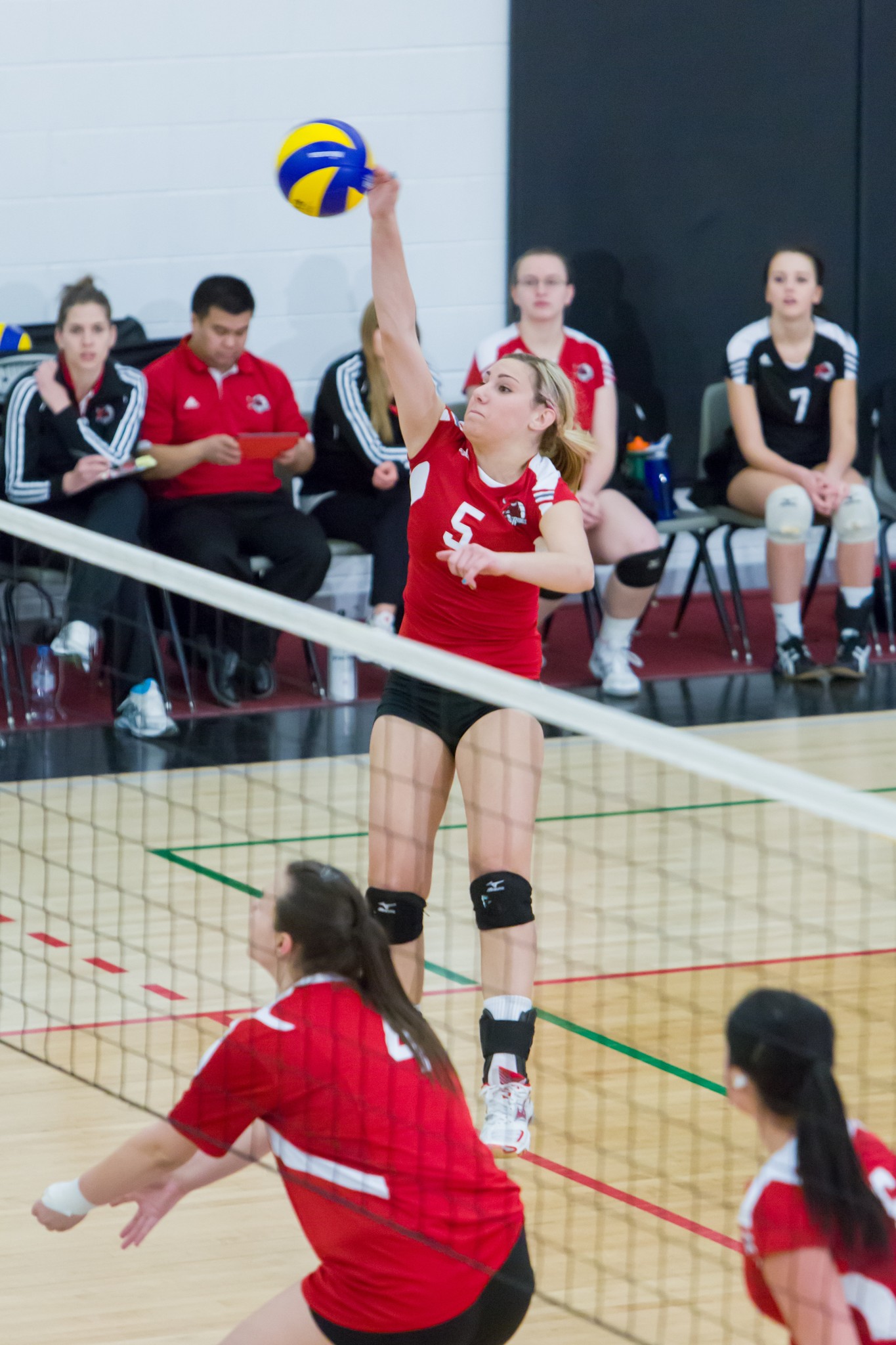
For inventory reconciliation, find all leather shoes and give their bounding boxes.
[204,648,240,707]
[242,656,273,698]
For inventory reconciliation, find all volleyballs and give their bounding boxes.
[275,119,372,217]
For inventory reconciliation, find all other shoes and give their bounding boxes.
[356,613,399,669]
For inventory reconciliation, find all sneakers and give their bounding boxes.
[477,1065,534,1161]
[588,636,642,697]
[50,619,96,673]
[114,677,177,738]
[831,634,871,677]
[772,637,823,680]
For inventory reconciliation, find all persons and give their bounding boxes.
[724,988,896,1345]
[28,858,535,1345]
[299,297,440,634]
[702,247,880,682]
[135,275,331,709]
[2,276,180,739]
[364,167,594,1159]
[461,244,666,699]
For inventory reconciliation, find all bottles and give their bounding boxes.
[328,610,358,703]
[644,450,673,519]
[627,435,649,484]
[29,645,55,723]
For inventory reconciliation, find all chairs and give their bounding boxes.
[0,404,468,731]
[582,508,738,659]
[668,383,881,667]
[872,457,896,655]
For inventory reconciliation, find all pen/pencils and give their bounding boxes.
[72,449,118,469]
